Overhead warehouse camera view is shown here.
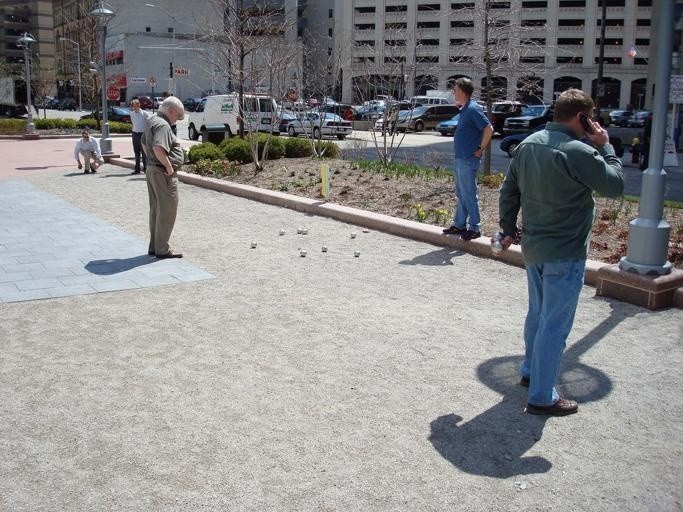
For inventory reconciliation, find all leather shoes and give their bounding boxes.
[520,374,531,388]
[148,248,183,259]
[527,398,578,416]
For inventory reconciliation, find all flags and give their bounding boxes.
[627,46,637,57]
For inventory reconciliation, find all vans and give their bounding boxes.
[187,91,281,143]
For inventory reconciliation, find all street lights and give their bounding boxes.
[59,37,82,113]
[87,1,121,158]
[16,31,41,137]
[144,2,176,78]
[15,44,42,113]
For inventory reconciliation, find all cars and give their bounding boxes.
[181,97,203,111]
[498,124,624,158]
[152,96,163,108]
[79,105,133,124]
[133,95,153,109]
[39,95,78,111]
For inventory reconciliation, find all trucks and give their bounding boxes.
[0,76,28,117]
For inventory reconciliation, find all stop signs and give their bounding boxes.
[105,85,120,101]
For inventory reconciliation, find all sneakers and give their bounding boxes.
[443,224,467,234]
[131,170,140,175]
[461,230,481,241]
[84,163,97,174]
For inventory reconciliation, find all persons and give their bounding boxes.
[443,77,494,240]
[381,110,391,136]
[390,109,399,136]
[638,113,652,171]
[130,99,150,175]
[145,95,185,258]
[492,88,625,416]
[74,131,105,174]
[159,92,176,137]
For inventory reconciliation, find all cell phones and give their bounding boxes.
[580,116,589,128]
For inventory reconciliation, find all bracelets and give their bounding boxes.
[478,145,484,151]
